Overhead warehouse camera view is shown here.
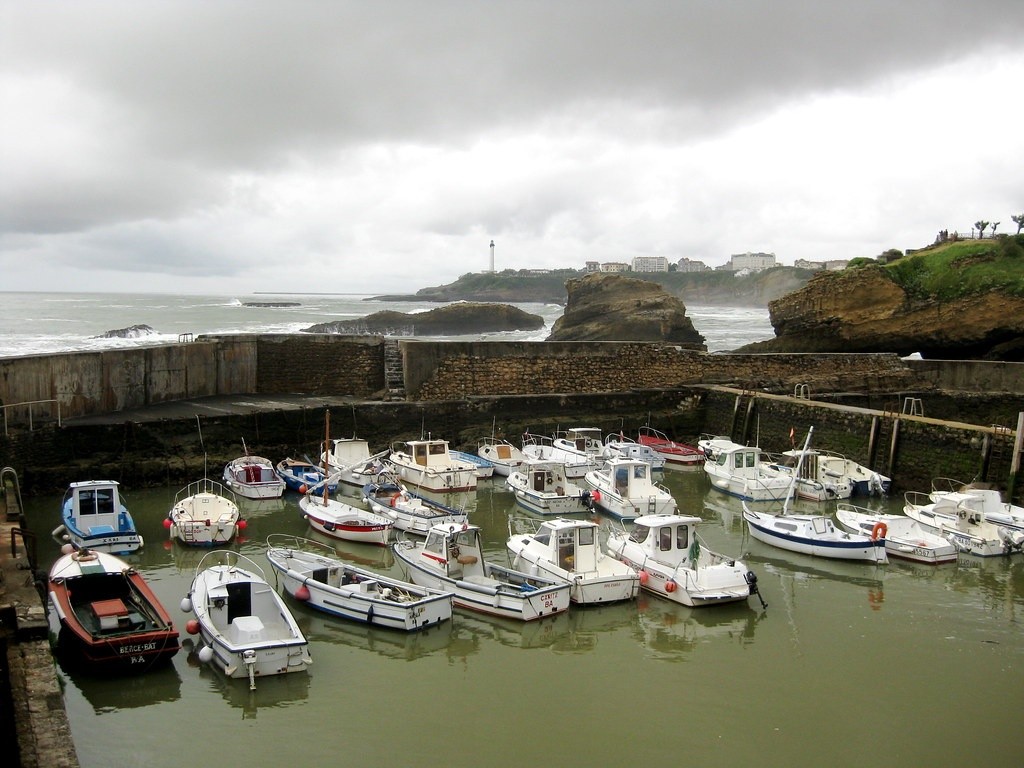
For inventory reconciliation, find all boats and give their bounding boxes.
[703,445,892,502]
[834,502,960,563]
[388,415,478,491]
[317,431,401,501]
[221,435,288,503]
[506,456,596,518]
[903,489,1024,558]
[262,533,455,630]
[928,477,1024,535]
[583,454,677,521]
[277,455,339,497]
[45,545,181,678]
[740,495,891,567]
[389,518,574,621]
[474,415,529,475]
[636,424,709,465]
[447,448,496,480]
[518,426,668,479]
[604,511,771,611]
[59,479,147,555]
[503,515,641,604]
[162,477,247,549]
[180,549,314,692]
[359,462,471,541]
[697,438,747,456]
[297,408,395,546]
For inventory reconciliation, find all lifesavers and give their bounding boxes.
[391,493,406,507]
[71,551,98,560]
[872,522,887,539]
